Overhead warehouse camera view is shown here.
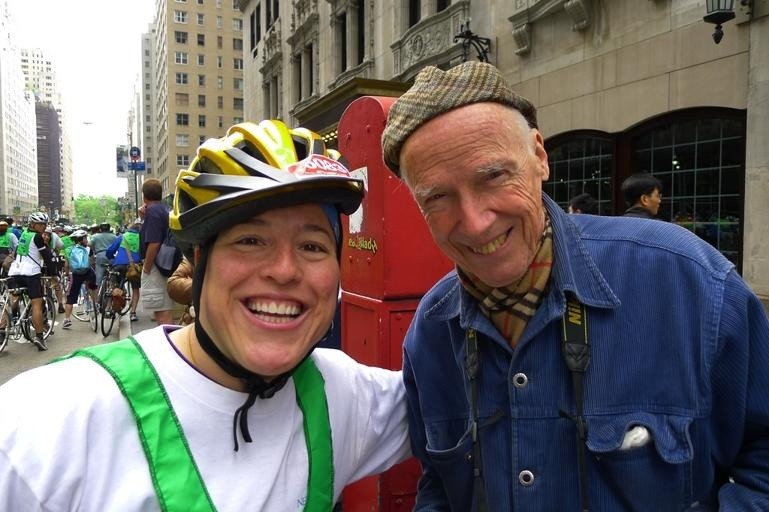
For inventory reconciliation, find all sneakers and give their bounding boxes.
[0,331,9,346]
[33,336,48,350]
[62,318,72,327]
[129,312,139,321]
[58,307,65,313]
[42,319,54,335]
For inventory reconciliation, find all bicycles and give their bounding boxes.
[0,263,133,356]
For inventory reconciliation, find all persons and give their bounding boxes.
[1,212,147,349]
[566,193,600,216]
[1,117,422,511]
[619,171,663,218]
[138,178,177,328]
[382,62,768,509]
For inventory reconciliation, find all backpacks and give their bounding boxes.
[140,206,183,276]
[68,244,90,276]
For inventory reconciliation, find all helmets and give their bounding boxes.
[44,220,114,239]
[0,216,23,227]
[27,211,48,226]
[157,115,367,245]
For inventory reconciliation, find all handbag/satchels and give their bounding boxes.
[125,261,142,284]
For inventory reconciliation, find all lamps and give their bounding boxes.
[702,0,754,44]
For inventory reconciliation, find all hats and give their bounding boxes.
[377,57,538,181]
